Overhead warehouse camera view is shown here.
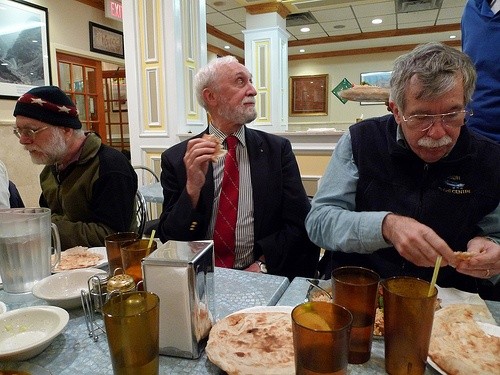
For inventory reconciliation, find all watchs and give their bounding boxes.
[256,260,268,274]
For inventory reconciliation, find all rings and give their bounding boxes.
[486,270,489,276]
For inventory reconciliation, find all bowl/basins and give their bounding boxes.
[0,305,70,360]
[31,267,109,308]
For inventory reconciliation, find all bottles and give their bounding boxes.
[105,275,136,301]
[91,273,108,308]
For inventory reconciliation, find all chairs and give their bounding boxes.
[127,189,147,239]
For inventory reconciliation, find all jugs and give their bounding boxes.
[0,207,61,308]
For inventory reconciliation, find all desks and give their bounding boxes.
[135,182,164,228]
[0,262,500,375]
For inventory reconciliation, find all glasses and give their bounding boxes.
[13,125,53,139]
[402,101,472,131]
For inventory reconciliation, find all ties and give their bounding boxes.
[213,135,240,269]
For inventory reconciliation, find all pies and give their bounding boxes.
[204,312,326,375]
[427,304,500,375]
[194,303,214,342]
[450,250,487,268]
[201,134,227,162]
[51,245,102,270]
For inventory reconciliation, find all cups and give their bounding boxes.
[120,239,158,291]
[102,291,161,375]
[382,276,438,375]
[291,301,353,375]
[104,231,140,276]
[331,265,380,364]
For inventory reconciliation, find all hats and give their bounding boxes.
[13,86,82,129]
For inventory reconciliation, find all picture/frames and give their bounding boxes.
[89,21,124,60]
[289,74,329,117]
[0,0,52,100]
[110,77,128,112]
[359,70,396,106]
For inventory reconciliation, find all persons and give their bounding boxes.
[385,0,500,159]
[13,84,138,255]
[305,42,500,302]
[0,160,10,209]
[157,55,320,283]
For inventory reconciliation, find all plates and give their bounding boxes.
[0,301,7,316]
[427,322,500,375]
[307,288,384,339]
[224,305,294,314]
[50,247,109,273]
